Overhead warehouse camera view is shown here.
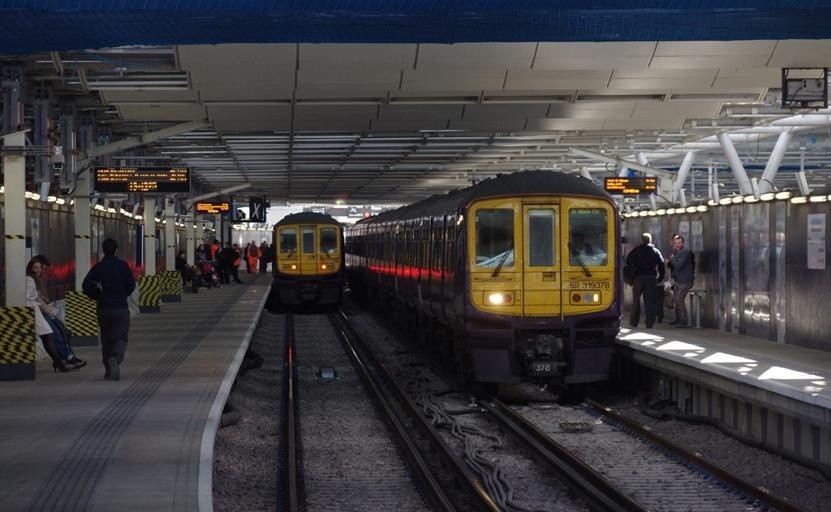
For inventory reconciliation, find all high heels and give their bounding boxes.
[53,357,86,370]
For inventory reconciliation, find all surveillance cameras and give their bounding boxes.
[51,154,65,175]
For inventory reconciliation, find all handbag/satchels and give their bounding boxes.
[86,279,102,300]
[623,266,634,285]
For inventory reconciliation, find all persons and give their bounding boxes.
[175,240,272,294]
[36,254,87,369]
[668,235,695,328]
[569,233,609,266]
[82,239,137,380]
[669,232,690,292]
[653,261,668,323]
[26,258,72,373]
[622,232,666,328]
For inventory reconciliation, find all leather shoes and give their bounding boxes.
[105,357,119,381]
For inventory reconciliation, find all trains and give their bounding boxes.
[344,164,624,403]
[268,211,347,307]
